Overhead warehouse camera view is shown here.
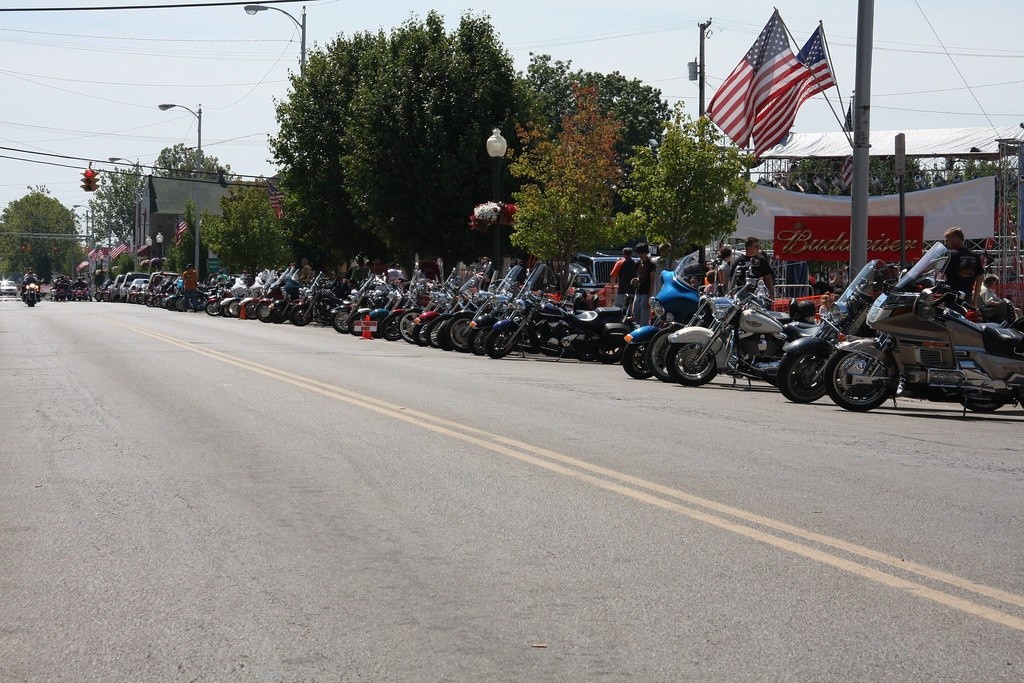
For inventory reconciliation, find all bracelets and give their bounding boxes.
[768,292,774,295]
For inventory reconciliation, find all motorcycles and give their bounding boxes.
[49,279,92,301]
[823,242,1023,417]
[330,264,640,363]
[665,277,818,388]
[646,258,790,382]
[132,266,343,326]
[21,276,42,306]
[776,258,1018,412]
[621,255,736,380]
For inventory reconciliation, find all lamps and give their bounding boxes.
[757,172,1024,196]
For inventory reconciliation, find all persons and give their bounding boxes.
[290,257,312,282]
[50,275,92,302]
[819,291,834,315]
[980,274,1022,323]
[938,227,984,308]
[20,267,43,302]
[610,243,679,327]
[354,255,443,292]
[211,270,228,285]
[182,263,198,312]
[704,237,777,305]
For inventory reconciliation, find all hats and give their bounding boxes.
[186,264,192,268]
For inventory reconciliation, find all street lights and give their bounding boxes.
[146,232,163,271]
[244,5,306,81]
[159,104,203,278]
[73,205,94,250]
[486,127,508,269]
[108,157,139,272]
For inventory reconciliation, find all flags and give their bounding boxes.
[704,8,813,151]
[752,24,837,161]
[76,261,89,273]
[266,179,287,218]
[108,238,128,260]
[175,213,188,246]
[88,246,103,262]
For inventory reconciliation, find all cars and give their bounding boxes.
[0,281,17,296]
[94,272,180,303]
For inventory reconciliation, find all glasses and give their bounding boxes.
[946,238,952,243]
[749,245,760,248]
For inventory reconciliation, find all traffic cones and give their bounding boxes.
[358,315,375,341]
[238,303,248,320]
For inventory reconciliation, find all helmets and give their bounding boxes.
[799,301,815,316]
[574,288,587,299]
[28,267,33,271]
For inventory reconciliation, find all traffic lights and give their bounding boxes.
[80,170,100,191]
